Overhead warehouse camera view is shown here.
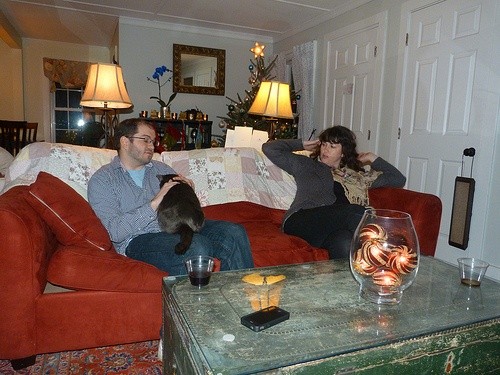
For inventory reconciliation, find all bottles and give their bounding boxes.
[349,208,420,307]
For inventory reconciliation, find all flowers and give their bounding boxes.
[152,65,167,80]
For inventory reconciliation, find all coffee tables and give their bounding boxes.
[162,254,500,375]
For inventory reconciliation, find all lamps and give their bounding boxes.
[242,273,288,311]
[246,81,294,137]
[79,63,134,147]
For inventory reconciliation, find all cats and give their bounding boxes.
[156,174,205,254]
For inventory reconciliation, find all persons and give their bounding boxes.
[88,119,254,282]
[262,125,406,261]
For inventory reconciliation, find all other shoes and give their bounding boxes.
[158,339,176,369]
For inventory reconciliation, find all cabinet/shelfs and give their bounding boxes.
[138,120,214,153]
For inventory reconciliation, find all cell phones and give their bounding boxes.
[240,305,290,332]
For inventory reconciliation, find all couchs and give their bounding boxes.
[0,141,443,361]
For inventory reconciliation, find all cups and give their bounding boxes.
[457,258,489,286]
[184,255,214,286]
[142,111,148,118]
[172,113,177,120]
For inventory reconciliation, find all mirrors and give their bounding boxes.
[172,44,226,96]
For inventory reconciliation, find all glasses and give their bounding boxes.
[125,137,159,147]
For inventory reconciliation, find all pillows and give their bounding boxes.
[26,165,113,251]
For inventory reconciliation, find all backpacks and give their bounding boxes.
[95,121,109,148]
